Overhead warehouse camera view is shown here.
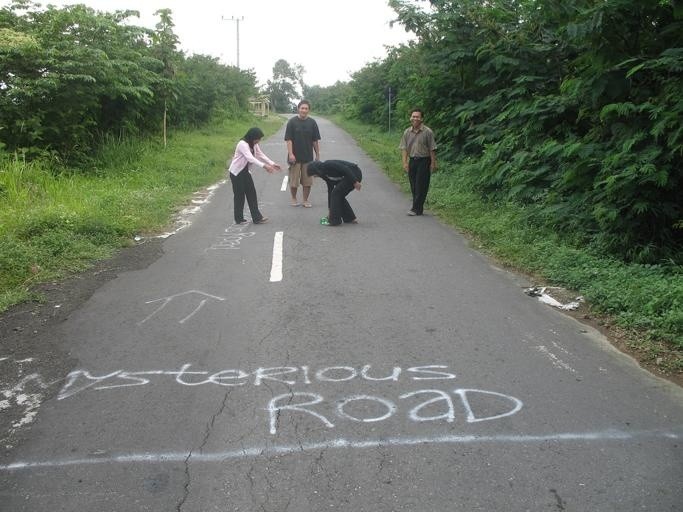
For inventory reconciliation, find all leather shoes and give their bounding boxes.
[236,217,268,225]
[407,207,423,216]
[327,219,357,225]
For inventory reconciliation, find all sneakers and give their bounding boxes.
[291,198,312,208]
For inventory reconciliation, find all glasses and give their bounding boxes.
[412,116,419,118]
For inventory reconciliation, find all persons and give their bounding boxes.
[306,161,363,225]
[227,128,282,224]
[284,100,320,208]
[398,109,438,217]
